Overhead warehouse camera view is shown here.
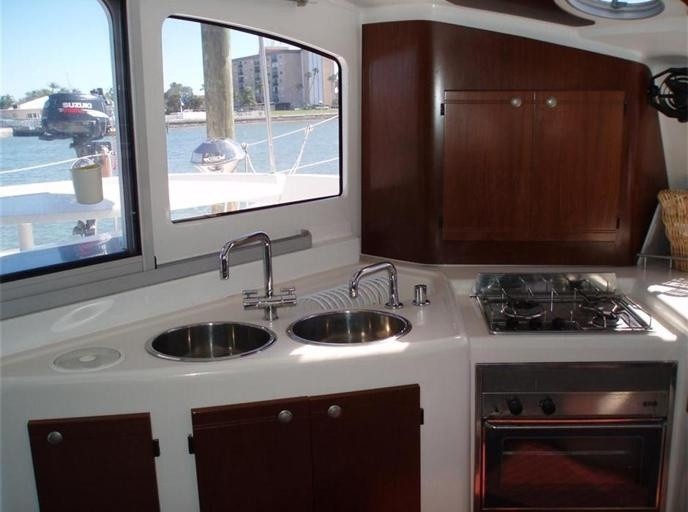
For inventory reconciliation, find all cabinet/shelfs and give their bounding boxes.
[25,414,159,512]
[191,385,424,509]
[444,90,627,246]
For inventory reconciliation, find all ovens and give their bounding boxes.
[473,361,676,510]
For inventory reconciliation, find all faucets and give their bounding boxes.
[218,232,275,319]
[347,260,401,310]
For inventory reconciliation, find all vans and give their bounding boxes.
[274,102,299,111]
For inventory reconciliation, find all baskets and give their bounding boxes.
[657,188,688,273]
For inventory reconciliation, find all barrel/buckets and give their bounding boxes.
[68,157,104,206]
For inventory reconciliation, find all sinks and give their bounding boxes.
[146,320,277,361]
[286,307,412,344]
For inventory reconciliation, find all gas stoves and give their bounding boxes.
[475,271,652,335]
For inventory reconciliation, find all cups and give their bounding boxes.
[69,164,104,206]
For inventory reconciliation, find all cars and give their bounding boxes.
[312,104,328,109]
